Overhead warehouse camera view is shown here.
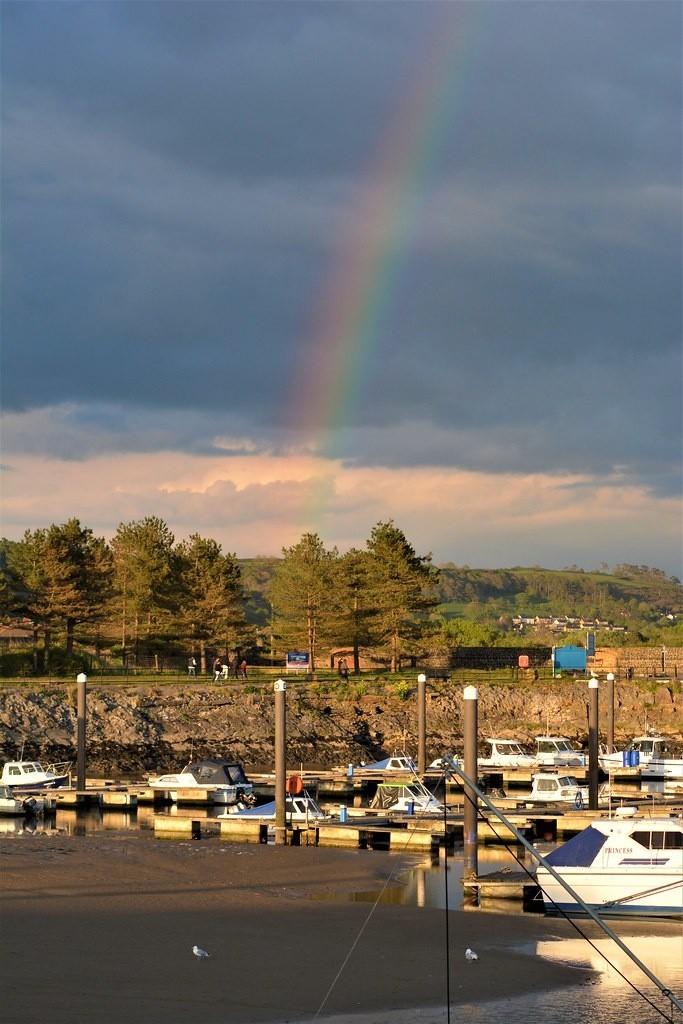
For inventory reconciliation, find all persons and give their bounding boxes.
[231,656,239,679]
[337,658,350,680]
[187,656,197,680]
[241,661,248,681]
[211,658,224,683]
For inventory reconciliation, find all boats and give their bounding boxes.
[0,761,72,789]
[328,779,451,813]
[489,773,612,805]
[598,736,683,778]
[147,759,258,804]
[362,757,417,770]
[534,806,683,919]
[429,735,589,767]
[218,797,333,820]
[0,782,45,816]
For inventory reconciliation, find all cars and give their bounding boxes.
[649,673,668,677]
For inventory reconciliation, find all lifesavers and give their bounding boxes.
[285,776,304,794]
